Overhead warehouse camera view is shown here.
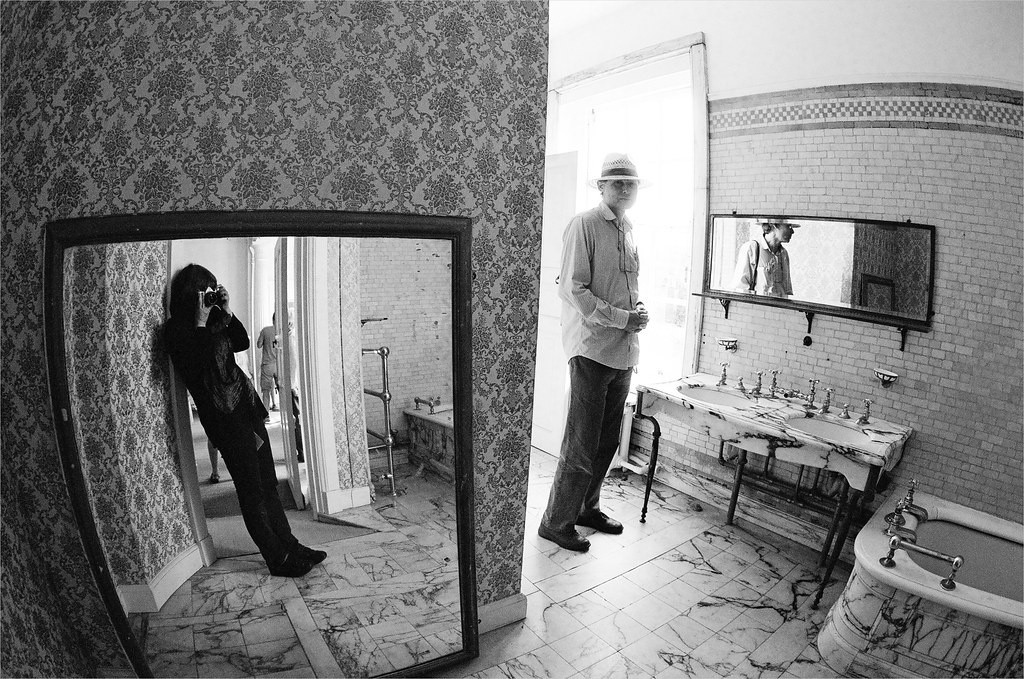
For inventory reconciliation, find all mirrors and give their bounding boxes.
[42,210,481,679]
[707,214,935,333]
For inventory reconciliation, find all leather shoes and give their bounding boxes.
[576,508,623,534]
[538,524,591,551]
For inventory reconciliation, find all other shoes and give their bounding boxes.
[263,416,270,423]
[273,404,279,411]
[270,551,312,577]
[210,473,219,484]
[286,543,328,564]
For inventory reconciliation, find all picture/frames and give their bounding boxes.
[861,273,896,312]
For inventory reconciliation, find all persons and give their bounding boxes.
[537,152,649,550]
[163,263,328,577]
[207,440,220,484]
[257,312,278,422]
[733,219,801,298]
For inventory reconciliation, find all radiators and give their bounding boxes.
[531,360,649,478]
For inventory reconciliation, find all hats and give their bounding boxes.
[756,217,801,228]
[597,152,639,180]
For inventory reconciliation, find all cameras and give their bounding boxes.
[199,288,224,307]
